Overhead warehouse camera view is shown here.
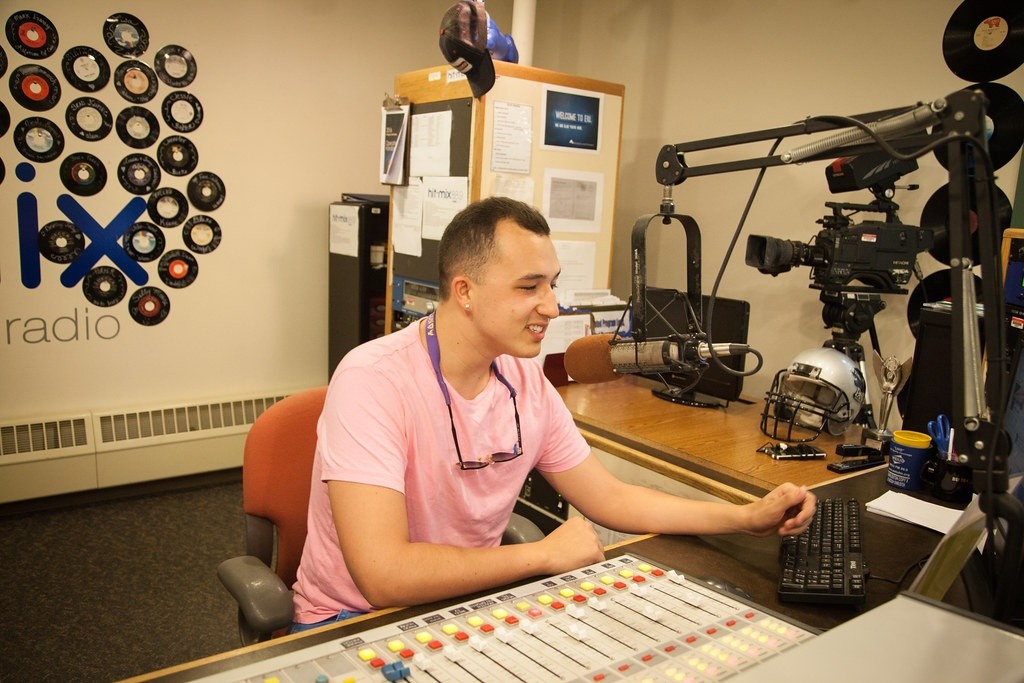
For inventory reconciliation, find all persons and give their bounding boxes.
[290,197,817,633]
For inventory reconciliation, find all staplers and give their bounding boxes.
[826,444,887,474]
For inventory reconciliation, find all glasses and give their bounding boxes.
[448,393,523,470]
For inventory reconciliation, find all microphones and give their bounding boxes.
[565,332,751,385]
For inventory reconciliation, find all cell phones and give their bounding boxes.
[766,446,827,460]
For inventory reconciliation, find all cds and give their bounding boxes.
[0,11,225,325]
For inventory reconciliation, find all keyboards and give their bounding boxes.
[778,498,872,605]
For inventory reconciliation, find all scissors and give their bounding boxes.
[928,413,950,458]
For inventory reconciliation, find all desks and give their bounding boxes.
[546,373,941,575]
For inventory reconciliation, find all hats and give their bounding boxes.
[439,0,496,98]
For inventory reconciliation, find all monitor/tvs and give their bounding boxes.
[633,286,751,407]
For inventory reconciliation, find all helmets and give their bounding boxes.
[773,346,866,436]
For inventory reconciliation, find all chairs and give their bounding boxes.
[216,385,545,646]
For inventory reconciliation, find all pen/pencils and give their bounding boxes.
[945,428,955,461]
